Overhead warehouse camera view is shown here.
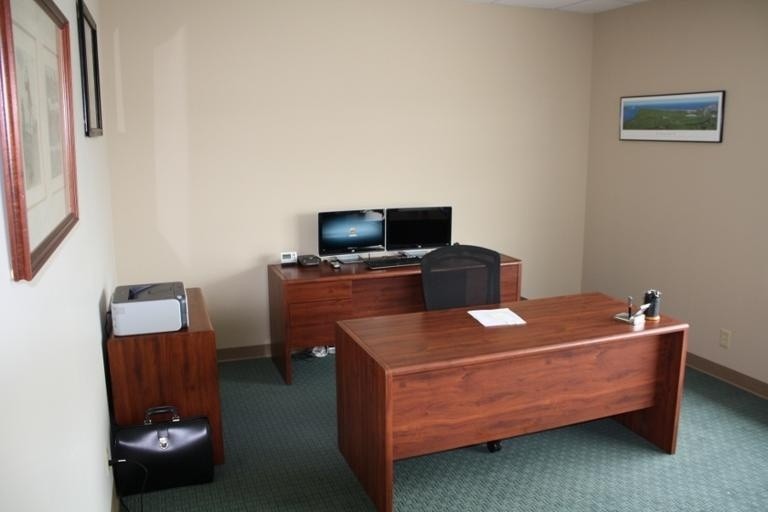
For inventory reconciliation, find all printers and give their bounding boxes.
[111,282,189,336]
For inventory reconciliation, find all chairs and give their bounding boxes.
[419,242,504,452]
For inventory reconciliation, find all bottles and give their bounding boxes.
[643,290,661,320]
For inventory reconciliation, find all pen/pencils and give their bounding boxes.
[647,289,660,297]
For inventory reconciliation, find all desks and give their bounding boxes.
[331,289,693,511]
[266,246,524,387]
[104,286,228,466]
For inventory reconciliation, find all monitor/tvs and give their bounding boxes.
[385,207,452,257]
[318,209,385,264]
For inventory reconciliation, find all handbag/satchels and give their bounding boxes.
[110,404,215,498]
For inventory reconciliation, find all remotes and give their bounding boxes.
[330,261,340,269]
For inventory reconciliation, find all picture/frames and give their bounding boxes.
[617,88,726,143]
[0,0,81,283]
[75,0,105,138]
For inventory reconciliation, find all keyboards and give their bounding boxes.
[366,257,421,270]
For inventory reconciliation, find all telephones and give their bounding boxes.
[298,255,320,266]
